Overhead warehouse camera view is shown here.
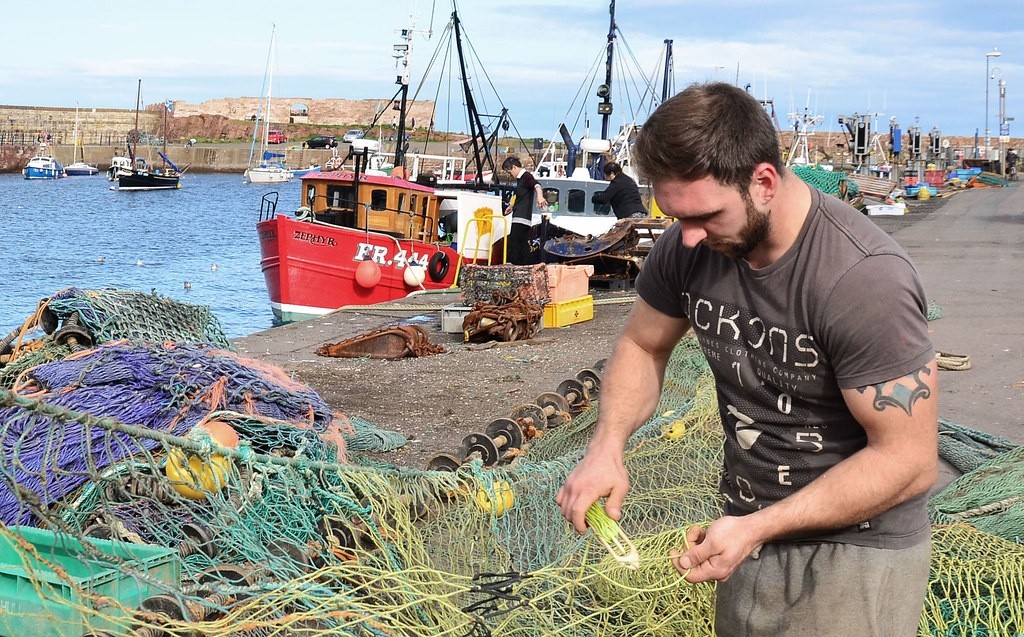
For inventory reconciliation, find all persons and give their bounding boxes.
[592,162,648,220]
[502,156,549,264]
[1006,148,1019,168]
[557,80,939,637]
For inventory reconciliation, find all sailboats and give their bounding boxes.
[260,32,322,178]
[62,101,99,177]
[243,23,295,184]
[114,78,193,191]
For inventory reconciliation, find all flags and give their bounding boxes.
[166,98,173,110]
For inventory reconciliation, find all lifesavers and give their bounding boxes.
[428,251,450,281]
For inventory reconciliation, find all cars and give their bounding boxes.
[267,129,287,144]
[301,135,339,150]
[342,129,365,143]
[385,133,411,142]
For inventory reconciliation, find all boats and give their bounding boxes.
[21,117,64,179]
[106,135,149,180]
[254,0,1024,324]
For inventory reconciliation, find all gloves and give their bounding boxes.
[592,196,600,203]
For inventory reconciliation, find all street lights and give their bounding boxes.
[984,51,1003,159]
